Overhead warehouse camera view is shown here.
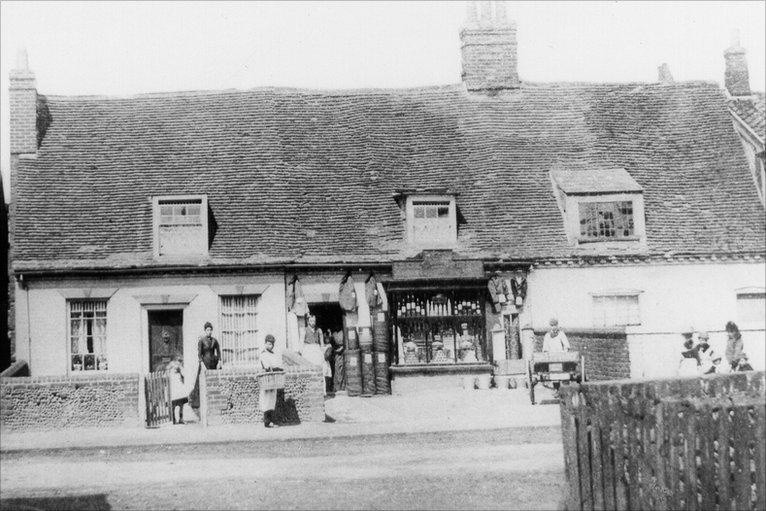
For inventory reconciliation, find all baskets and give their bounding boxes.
[255,371,286,389]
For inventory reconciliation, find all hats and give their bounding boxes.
[266,335,276,342]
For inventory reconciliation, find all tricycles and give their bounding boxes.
[523,349,586,406]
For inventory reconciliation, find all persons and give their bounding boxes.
[258,334,286,428]
[676,320,755,377]
[542,318,570,399]
[198,322,222,373]
[298,314,345,400]
[164,352,188,425]
